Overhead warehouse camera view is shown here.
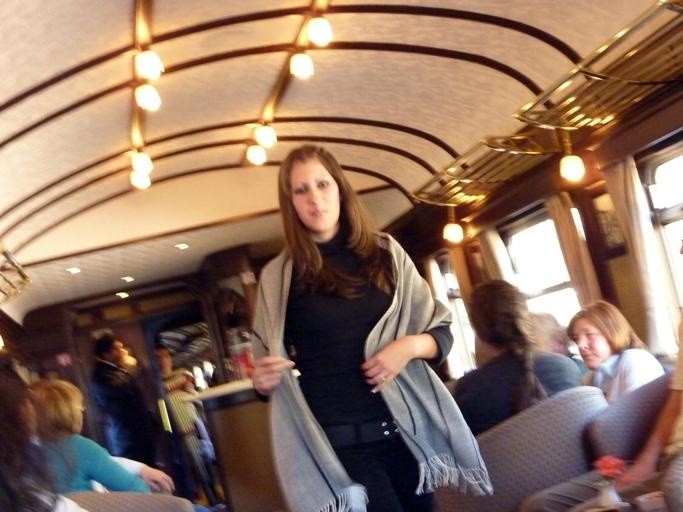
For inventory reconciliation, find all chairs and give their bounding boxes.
[584,358,681,476]
[56,488,209,512]
[425,376,609,509]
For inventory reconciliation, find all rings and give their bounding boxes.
[382,374,388,382]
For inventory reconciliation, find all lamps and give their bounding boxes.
[558,135,587,185]
[441,201,464,248]
[122,13,338,189]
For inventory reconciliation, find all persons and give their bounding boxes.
[432,359,456,393]
[0,361,214,512]
[566,301,665,404]
[86,332,227,512]
[249,145,493,511]
[534,314,590,375]
[451,279,582,438]
[474,334,504,368]
[521,248,683,512]
